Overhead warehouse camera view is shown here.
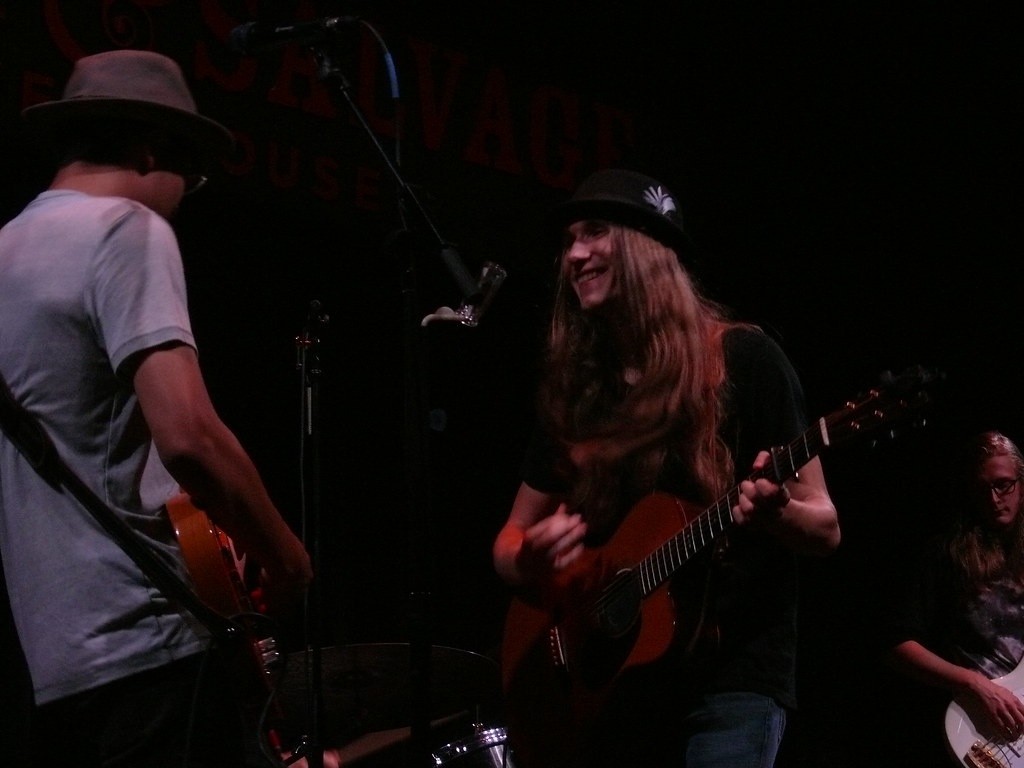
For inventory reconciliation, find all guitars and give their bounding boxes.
[503,359,936,745]
[159,488,271,642]
[942,652,1024,768]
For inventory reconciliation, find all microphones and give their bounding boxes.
[230,15,364,55]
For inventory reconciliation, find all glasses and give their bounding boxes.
[980,475,1018,500]
[188,174,207,192]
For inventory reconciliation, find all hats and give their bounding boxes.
[21,50,235,176]
[559,168,695,253]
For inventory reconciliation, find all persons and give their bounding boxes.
[493,170,841,768]
[864,432,1024,768]
[0,50,312,768]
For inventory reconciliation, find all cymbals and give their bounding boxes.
[280,641,502,729]
